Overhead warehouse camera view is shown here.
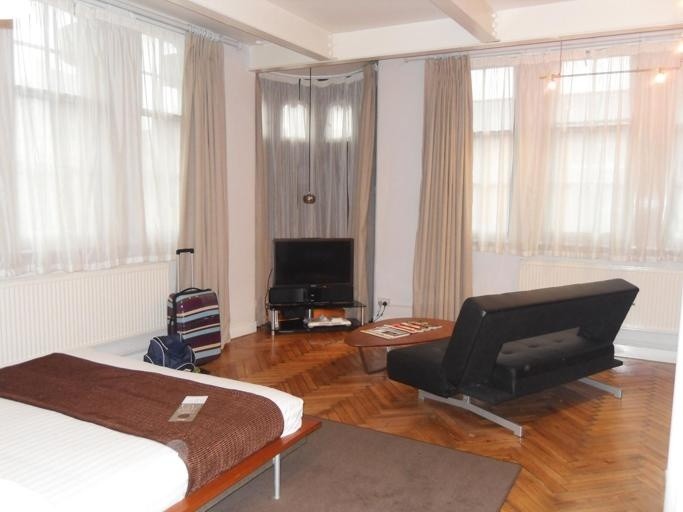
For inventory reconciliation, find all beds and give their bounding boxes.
[0,350,322,512]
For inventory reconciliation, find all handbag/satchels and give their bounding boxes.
[143,336,198,373]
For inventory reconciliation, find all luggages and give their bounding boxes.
[166,248,221,367]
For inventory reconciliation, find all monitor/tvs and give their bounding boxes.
[273,237,354,302]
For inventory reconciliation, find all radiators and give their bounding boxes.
[0,260,168,364]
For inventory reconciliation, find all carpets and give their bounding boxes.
[206,420,521,511]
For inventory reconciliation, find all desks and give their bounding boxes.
[268,301,366,336]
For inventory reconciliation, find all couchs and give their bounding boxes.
[384,277,639,436]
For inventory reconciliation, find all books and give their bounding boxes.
[359,319,442,340]
